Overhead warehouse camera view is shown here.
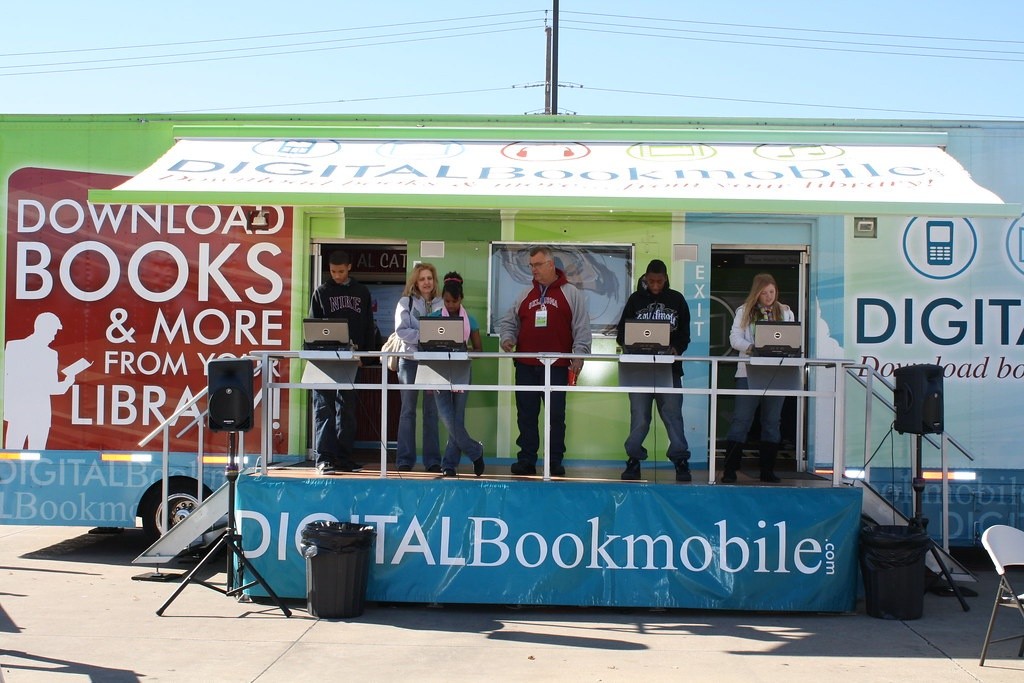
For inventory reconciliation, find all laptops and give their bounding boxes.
[623,320,674,355]
[749,321,801,358]
[418,316,468,352]
[302,318,352,351]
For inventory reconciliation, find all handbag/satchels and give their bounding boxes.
[380,296,413,371]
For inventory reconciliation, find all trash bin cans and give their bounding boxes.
[860,524,929,621]
[302,520,375,618]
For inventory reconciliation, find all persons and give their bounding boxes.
[616,260,692,481]
[721,274,795,483]
[500,246,592,476]
[394,263,444,472]
[307,251,376,474]
[426,272,485,476]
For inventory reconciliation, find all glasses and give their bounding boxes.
[528,259,549,269]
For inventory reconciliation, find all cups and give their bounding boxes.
[568,366,581,386]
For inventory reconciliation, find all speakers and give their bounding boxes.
[894,364,944,435]
[208,358,254,432]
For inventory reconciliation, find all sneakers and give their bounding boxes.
[675,460,693,482]
[620,458,641,480]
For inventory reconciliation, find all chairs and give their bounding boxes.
[979,525,1024,666]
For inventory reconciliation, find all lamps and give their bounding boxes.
[855,216,877,240]
[247,210,271,231]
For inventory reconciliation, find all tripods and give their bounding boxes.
[155,432,292,618]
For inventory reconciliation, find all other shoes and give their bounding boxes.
[549,463,565,477]
[472,446,485,476]
[511,461,536,476]
[429,464,441,473]
[334,461,363,472]
[399,464,411,472]
[759,470,781,484]
[317,461,335,474]
[444,468,456,477]
[721,464,737,484]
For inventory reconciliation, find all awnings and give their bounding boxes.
[87,137,1022,217]
